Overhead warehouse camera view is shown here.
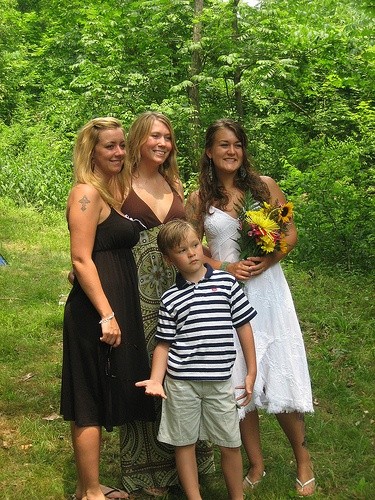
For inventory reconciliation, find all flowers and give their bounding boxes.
[229,187,294,290]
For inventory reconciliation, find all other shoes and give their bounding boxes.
[143,488,170,498]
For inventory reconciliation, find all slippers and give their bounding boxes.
[243,471,266,492]
[104,486,129,499]
[296,478,316,498]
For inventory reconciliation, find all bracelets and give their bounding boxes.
[99,312,114,324]
[220,261,230,271]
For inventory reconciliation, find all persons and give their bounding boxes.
[67,113,216,499]
[134,217,258,500]
[183,119,317,498]
[60,117,159,500]
